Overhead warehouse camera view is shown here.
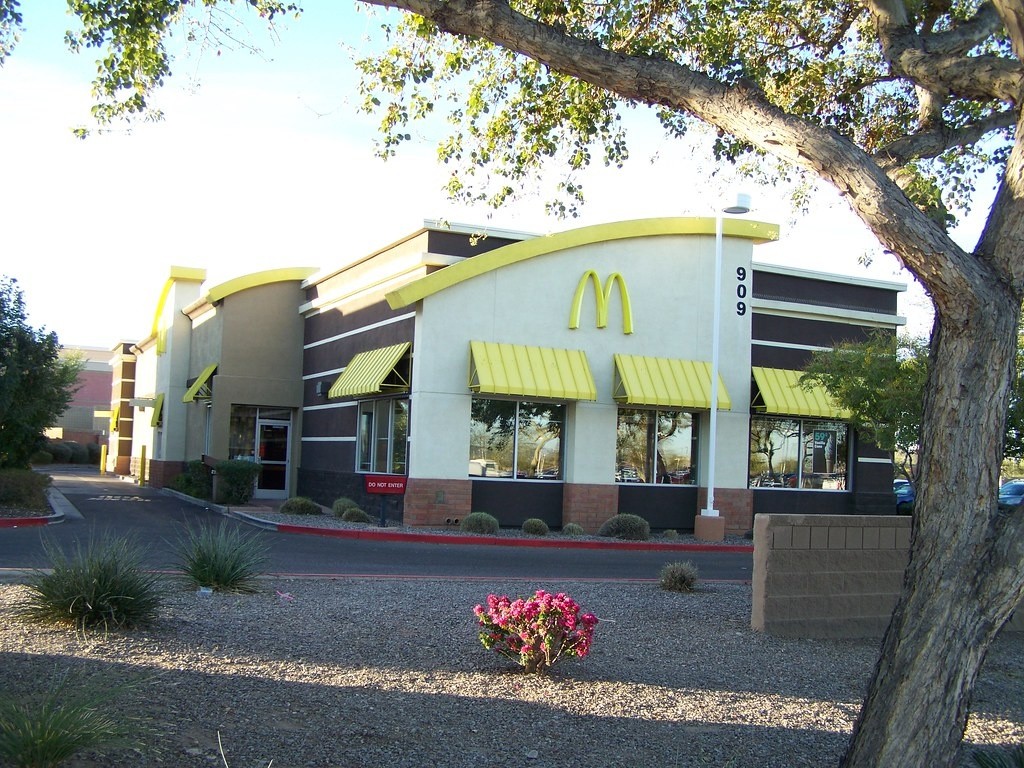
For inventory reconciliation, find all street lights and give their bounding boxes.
[694,191,751,541]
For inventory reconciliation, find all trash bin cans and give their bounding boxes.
[211,469,227,503]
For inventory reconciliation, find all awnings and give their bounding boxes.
[468,342,597,402]
[612,354,730,413]
[326,341,413,398]
[752,366,877,421]
[183,363,217,402]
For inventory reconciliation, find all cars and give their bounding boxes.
[999,482,1023,508]
[894,479,915,515]
[517,468,559,479]
[615,467,692,484]
[748,470,847,491]
